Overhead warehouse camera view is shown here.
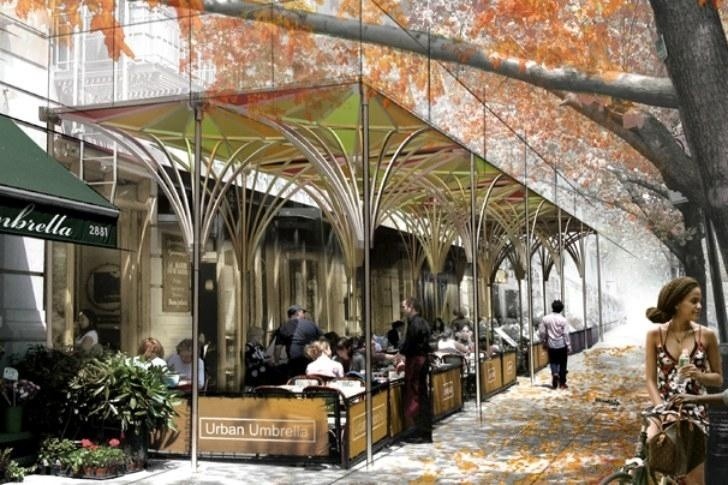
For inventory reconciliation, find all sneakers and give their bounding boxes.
[403,426,433,444]
[552,373,568,389]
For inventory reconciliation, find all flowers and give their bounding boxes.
[0,378,40,407]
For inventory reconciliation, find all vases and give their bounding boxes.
[6,406,22,433]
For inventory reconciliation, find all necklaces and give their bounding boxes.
[669,324,692,344]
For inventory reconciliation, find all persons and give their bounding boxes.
[386,319,405,350]
[127,336,171,375]
[75,308,100,354]
[166,339,205,393]
[391,296,434,444]
[244,326,272,389]
[273,304,325,386]
[430,308,543,365]
[304,332,394,378]
[622,275,724,485]
[668,390,728,408]
[539,299,572,389]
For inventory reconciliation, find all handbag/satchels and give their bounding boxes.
[648,419,705,476]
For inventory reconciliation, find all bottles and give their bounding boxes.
[676,348,690,386]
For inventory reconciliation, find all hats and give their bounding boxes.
[288,306,309,318]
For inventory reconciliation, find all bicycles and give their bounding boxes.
[595,396,709,485]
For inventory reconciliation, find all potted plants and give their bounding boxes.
[37,437,126,479]
[58,349,182,474]
